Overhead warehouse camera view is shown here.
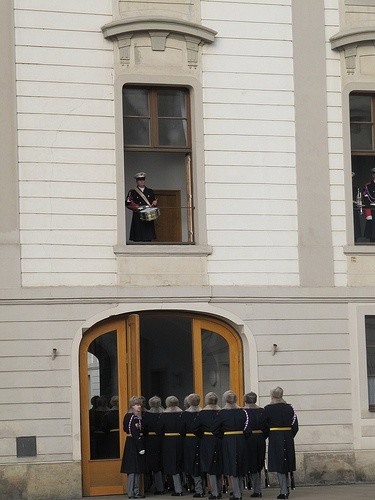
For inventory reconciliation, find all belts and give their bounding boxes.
[224,431,243,435]
[204,432,213,435]
[270,427,291,430]
[110,429,119,431]
[126,432,196,436]
[93,431,104,433]
[252,430,263,433]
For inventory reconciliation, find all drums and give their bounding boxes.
[141,208,161,221]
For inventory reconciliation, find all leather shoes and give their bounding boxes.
[277,493,289,499]
[128,495,145,499]
[251,492,262,498]
[167,485,228,495]
[171,492,183,496]
[192,493,204,498]
[153,491,163,495]
[208,495,222,499]
[229,493,243,500]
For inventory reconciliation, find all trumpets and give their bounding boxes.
[355,186,362,215]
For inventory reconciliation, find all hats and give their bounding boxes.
[184,394,201,407]
[166,396,179,407]
[148,396,162,408]
[271,386,284,397]
[223,390,237,403]
[135,172,145,180]
[91,396,119,407]
[129,396,146,406]
[205,392,218,405]
[243,391,257,403]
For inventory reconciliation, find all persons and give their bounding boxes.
[163,395,187,496]
[362,168,375,243]
[119,396,147,498]
[241,391,270,498]
[148,396,167,495]
[203,392,223,500]
[108,395,121,458]
[125,172,160,246]
[183,394,205,499]
[263,385,299,500]
[88,395,110,459]
[219,390,247,500]
